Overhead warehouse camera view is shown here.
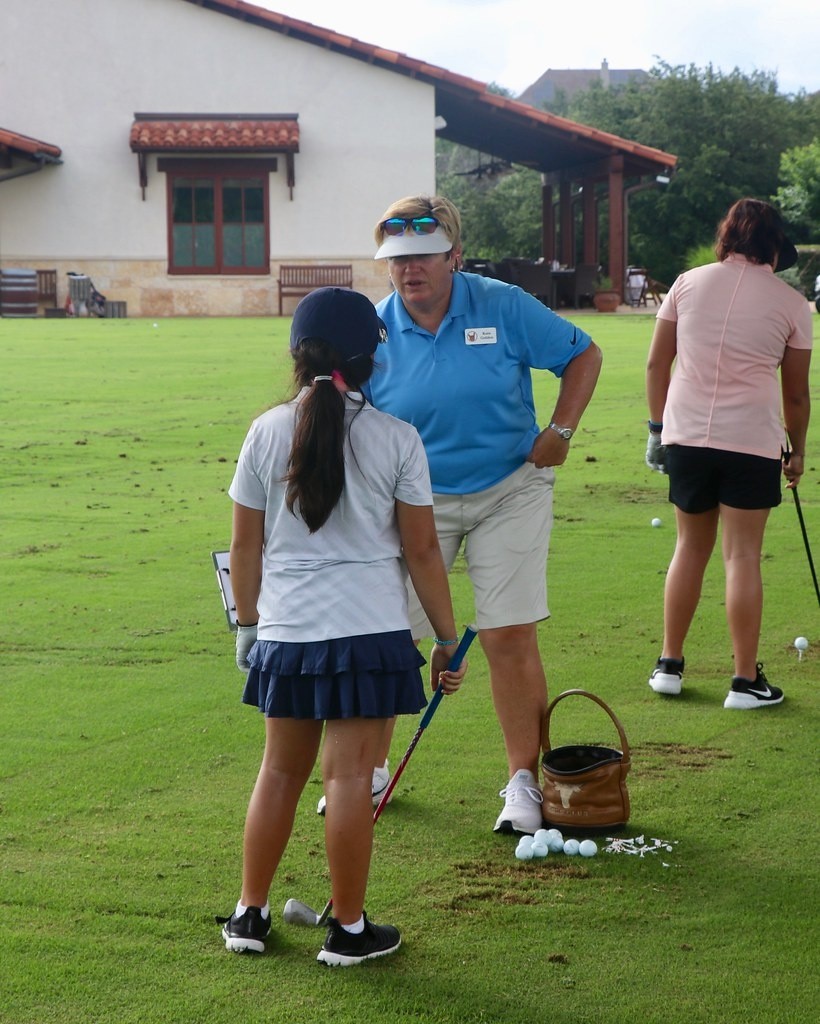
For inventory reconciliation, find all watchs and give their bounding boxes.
[549,422,575,440]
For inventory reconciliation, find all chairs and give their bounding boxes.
[460,256,600,311]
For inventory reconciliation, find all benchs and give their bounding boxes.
[277,264,353,315]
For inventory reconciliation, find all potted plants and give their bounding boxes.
[591,276,620,313]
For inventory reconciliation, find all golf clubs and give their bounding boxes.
[282,624,480,928]
[780,425,820,606]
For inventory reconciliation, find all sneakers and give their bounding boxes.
[317,759,393,814]
[723,662,784,709]
[493,769,543,835]
[317,910,402,968]
[649,655,684,694]
[215,906,271,952]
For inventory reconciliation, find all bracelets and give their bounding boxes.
[433,636,459,645]
[648,421,663,432]
[791,451,805,457]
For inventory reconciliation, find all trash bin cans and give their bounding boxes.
[69,276,92,316]
[625,267,647,304]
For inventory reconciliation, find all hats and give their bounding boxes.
[289,286,388,355]
[374,226,453,260]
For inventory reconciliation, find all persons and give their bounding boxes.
[222,287,469,966]
[316,196,602,835]
[646,198,812,709]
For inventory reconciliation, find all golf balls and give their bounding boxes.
[515,828,598,860]
[651,518,661,527]
[795,637,808,650]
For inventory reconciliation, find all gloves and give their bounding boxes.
[646,430,665,474]
[235,619,258,672]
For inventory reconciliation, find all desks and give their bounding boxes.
[550,268,575,309]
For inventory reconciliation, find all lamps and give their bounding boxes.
[461,144,516,189]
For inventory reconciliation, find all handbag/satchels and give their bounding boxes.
[541,689,631,829]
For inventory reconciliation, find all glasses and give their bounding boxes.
[379,218,438,235]
